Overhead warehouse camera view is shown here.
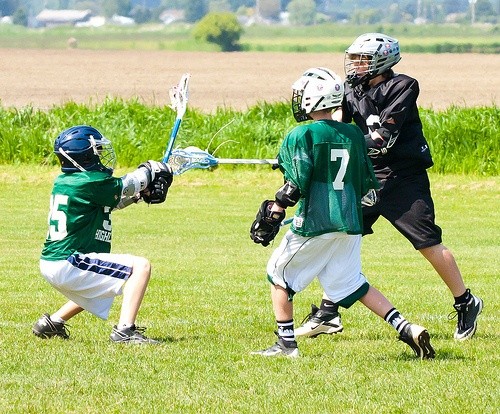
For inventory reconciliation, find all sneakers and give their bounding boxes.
[399,323,435,359]
[32,313,70,339]
[110,324,157,343]
[447,288,483,340]
[251,331,299,357]
[294,304,343,338]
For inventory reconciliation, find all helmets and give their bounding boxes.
[292,67,345,122]
[344,33,402,89]
[53,125,116,176]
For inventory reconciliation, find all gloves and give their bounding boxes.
[249,200,285,247]
[138,160,173,185]
[141,178,170,204]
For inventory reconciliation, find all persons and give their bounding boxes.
[293,33,484,341]
[33,126,173,343]
[250,67,438,359]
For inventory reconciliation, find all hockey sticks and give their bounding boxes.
[162,72,192,162]
[167,145,280,176]
